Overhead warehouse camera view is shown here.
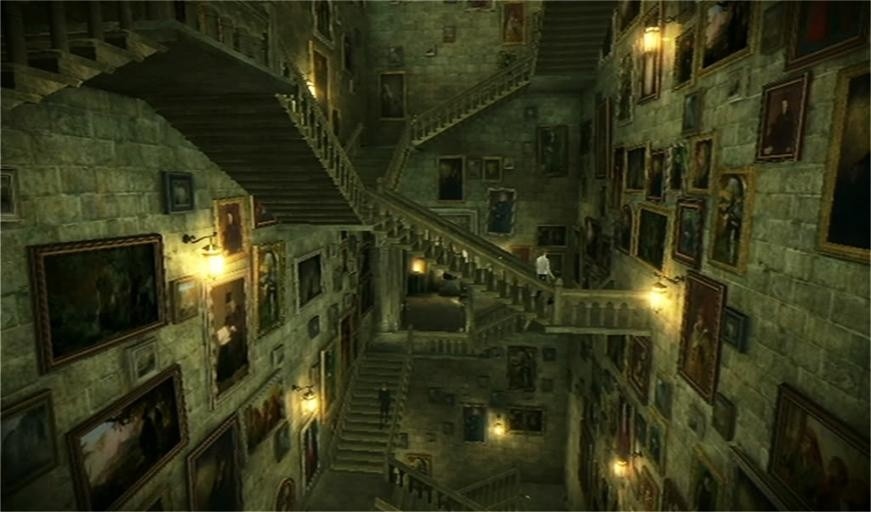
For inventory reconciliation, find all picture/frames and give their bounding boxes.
[574,2,869,507]
[340,2,573,491]
[3,3,338,509]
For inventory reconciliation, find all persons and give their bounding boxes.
[691,306,711,386]
[215,292,246,394]
[824,455,853,511]
[791,426,824,503]
[505,9,522,42]
[244,394,281,454]
[535,249,555,305]
[679,210,695,257]
[222,211,243,256]
[693,470,713,511]
[377,382,390,429]
[692,143,710,189]
[763,98,794,155]
